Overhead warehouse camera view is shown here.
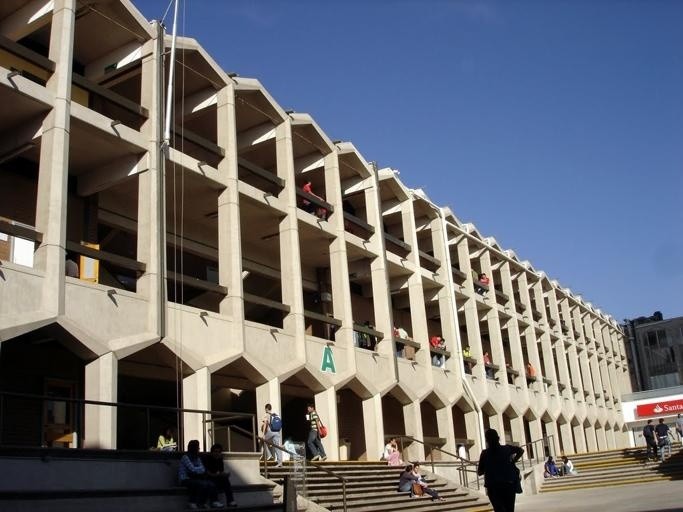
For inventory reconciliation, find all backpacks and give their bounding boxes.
[270,413,281,432]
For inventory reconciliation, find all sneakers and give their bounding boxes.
[647,457,658,461]
[200,504,209,509]
[227,501,237,507]
[275,463,282,467]
[311,455,319,461]
[432,498,446,501]
[212,502,224,507]
[319,457,327,461]
[267,455,272,460]
[186,502,197,509]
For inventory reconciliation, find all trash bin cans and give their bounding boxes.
[340,437,352,461]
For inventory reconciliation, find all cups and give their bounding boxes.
[305,415,309,420]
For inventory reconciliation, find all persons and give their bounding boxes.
[317,194,327,221]
[642,419,659,461]
[62,253,79,279]
[411,463,446,501]
[475,272,488,295]
[175,439,214,509]
[558,456,573,477]
[352,316,536,384]
[396,464,420,497]
[655,419,675,462]
[544,455,560,477]
[201,444,238,506]
[383,437,400,466]
[261,403,283,468]
[301,180,326,219]
[304,403,327,461]
[156,426,175,453]
[475,429,525,511]
[675,413,683,446]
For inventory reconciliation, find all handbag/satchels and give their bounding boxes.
[658,439,665,447]
[514,465,522,493]
[319,426,328,438]
[412,483,425,495]
